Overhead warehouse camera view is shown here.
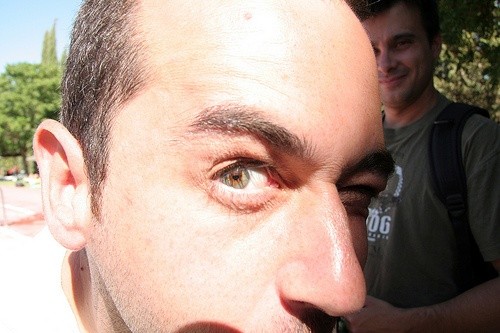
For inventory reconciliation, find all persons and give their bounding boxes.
[340,1,500,333]
[0,2,395,333]
